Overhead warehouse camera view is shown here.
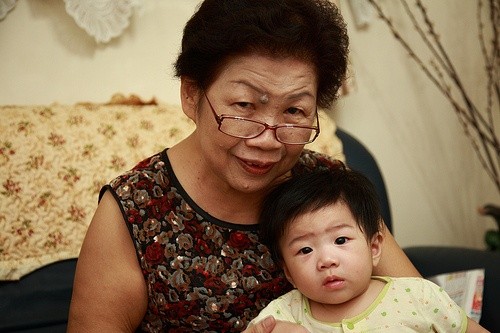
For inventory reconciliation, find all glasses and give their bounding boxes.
[200,83,321,145]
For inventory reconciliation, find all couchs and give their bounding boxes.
[0,104,500,333]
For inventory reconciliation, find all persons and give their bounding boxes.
[67,0,422,333]
[246,167,492,333]
